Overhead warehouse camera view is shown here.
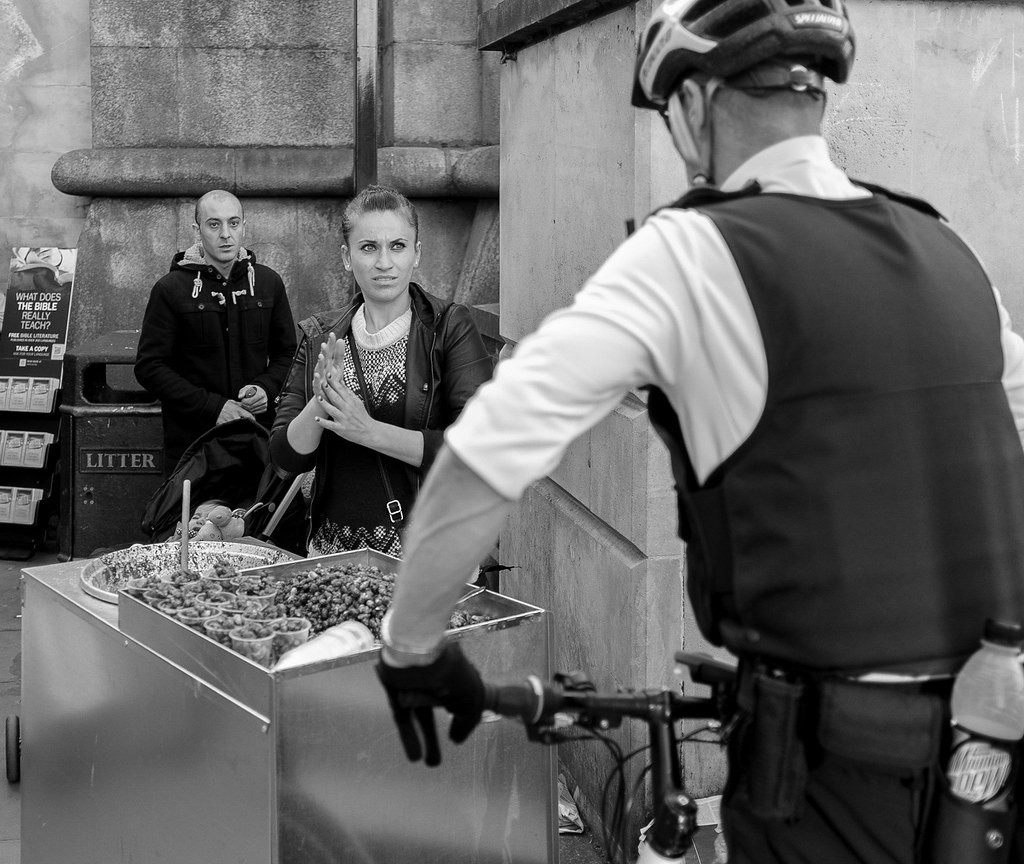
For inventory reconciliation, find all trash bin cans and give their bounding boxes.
[56,330,166,565]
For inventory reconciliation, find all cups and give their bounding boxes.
[126,571,313,673]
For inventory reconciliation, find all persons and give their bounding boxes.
[134,187,297,479]
[58,499,230,563]
[372,1,1024,864]
[267,182,494,590]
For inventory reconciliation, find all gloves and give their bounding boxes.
[376,637,488,768]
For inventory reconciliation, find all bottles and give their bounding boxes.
[934,619,1024,817]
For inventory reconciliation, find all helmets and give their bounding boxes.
[632,0,860,107]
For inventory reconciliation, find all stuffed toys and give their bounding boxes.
[190,506,245,542]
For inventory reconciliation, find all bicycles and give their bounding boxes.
[472,672,738,864]
